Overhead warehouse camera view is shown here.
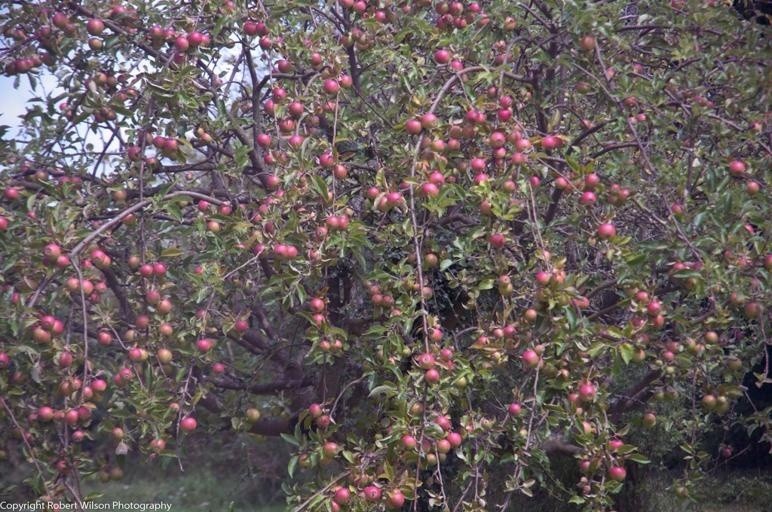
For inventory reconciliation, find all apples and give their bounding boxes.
[1,0,772,512]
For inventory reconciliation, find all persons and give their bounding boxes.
[403,264,480,491]
